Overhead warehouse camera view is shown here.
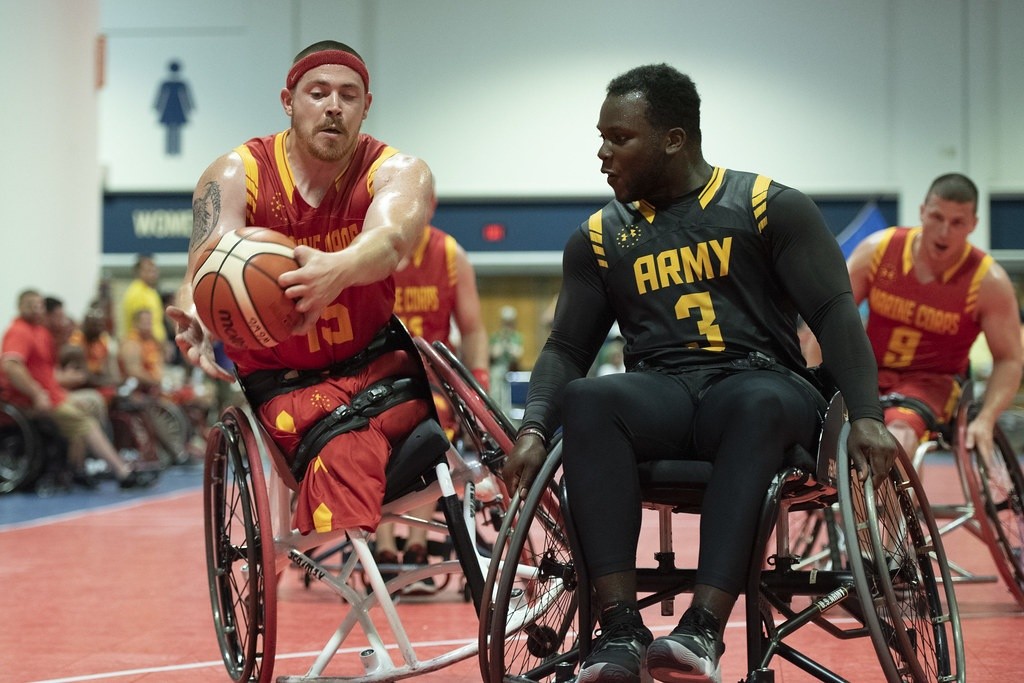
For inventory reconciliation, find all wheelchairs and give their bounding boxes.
[202,314,574,683]
[479,426,965,683]
[791,397,1023,610]
[0,390,192,496]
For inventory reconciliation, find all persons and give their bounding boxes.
[804,173,1022,604]
[494,63,902,683]
[190,39,494,529]
[0,252,221,492]
[358,226,493,605]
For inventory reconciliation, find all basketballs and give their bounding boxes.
[190,227,301,348]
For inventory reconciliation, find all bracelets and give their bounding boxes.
[514,429,550,446]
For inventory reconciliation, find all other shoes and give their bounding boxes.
[65,469,98,489]
[120,468,159,489]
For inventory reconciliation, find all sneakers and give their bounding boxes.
[649,604,726,682]
[401,545,437,593]
[579,601,655,683]
[373,550,399,607]
